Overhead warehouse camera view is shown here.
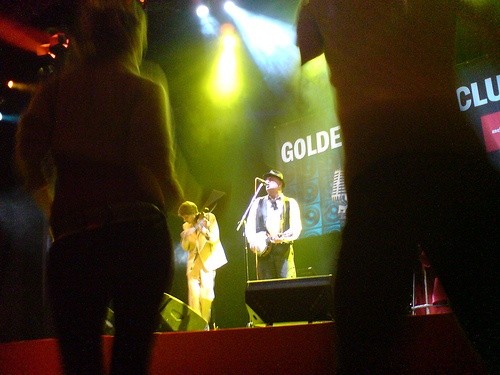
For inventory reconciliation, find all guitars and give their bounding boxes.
[180,207,209,251]
[251,231,291,255]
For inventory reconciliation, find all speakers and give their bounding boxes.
[156,291,208,332]
[102,305,116,335]
[245,274,335,326]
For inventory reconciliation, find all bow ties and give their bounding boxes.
[268,196,280,211]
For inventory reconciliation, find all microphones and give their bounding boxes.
[331,169,349,230]
[195,207,210,219]
[257,177,270,186]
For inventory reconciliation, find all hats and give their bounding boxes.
[262,169,285,184]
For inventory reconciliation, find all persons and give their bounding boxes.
[244,170,302,281]
[17,0,185,375]
[178,200,228,325]
[296,0,500,375]
